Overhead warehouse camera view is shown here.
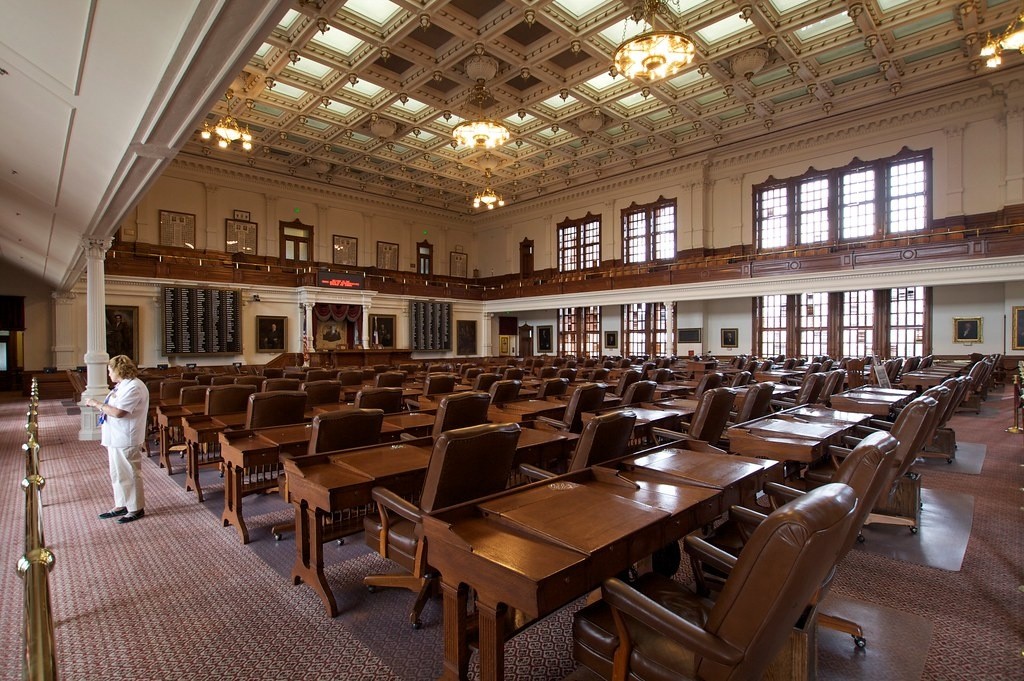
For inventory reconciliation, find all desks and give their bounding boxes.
[154,403,211,474]
[829,383,917,420]
[903,363,961,393]
[937,358,975,371]
[693,361,739,382]
[598,387,716,442]
[429,437,788,681]
[416,382,539,413]
[284,422,569,618]
[501,381,605,425]
[732,380,802,409]
[634,367,703,401]
[725,402,875,491]
[820,365,868,387]
[219,410,434,545]
[183,398,356,504]
[756,367,809,383]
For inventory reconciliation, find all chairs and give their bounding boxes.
[63,223,1006,681]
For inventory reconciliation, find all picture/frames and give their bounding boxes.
[369,313,397,349]
[106,304,141,366]
[536,325,554,352]
[604,330,619,349]
[720,327,739,348]
[952,316,983,345]
[678,328,701,343]
[457,320,478,356]
[255,315,290,353]
[1011,305,1024,350]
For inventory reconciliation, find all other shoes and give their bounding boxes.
[99,506,128,518]
[118,508,144,523]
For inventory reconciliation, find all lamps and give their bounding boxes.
[470,155,507,211]
[612,0,699,80]
[200,91,255,154]
[977,9,1024,72]
[449,56,511,152]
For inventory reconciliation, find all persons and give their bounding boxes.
[85,354,150,524]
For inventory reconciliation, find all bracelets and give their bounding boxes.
[99,404,106,412]
